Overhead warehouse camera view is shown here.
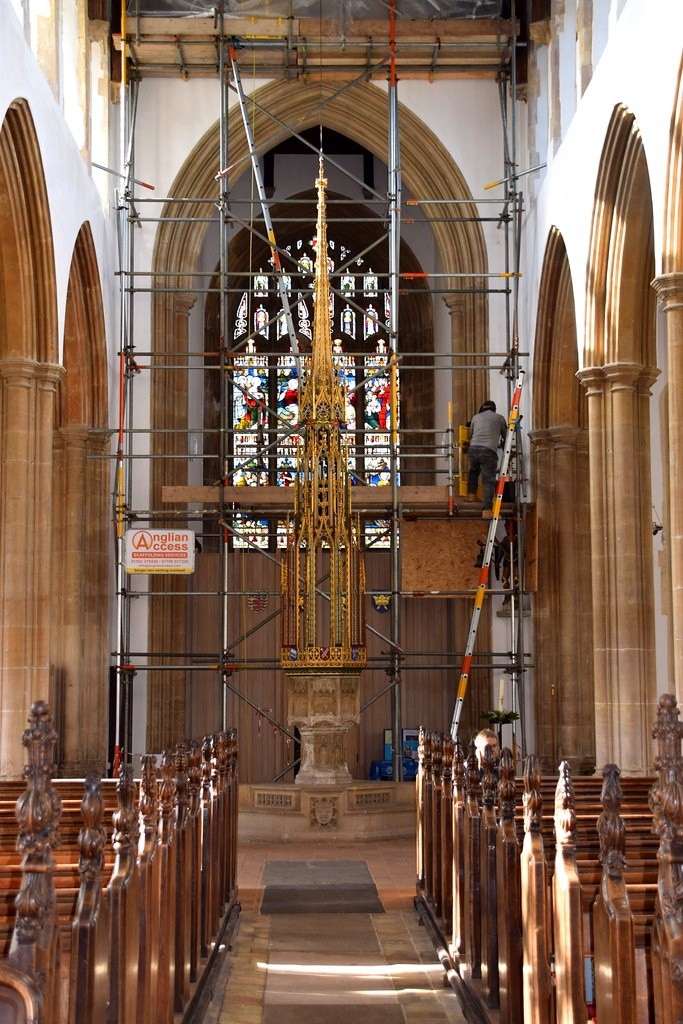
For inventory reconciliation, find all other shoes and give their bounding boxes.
[482,508,502,519]
[468,493,481,502]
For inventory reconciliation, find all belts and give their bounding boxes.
[470,445,490,450]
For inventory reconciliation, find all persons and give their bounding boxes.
[474,729,500,762]
[467,401,507,518]
[496,519,520,610]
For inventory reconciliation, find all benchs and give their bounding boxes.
[0,701,241,1024]
[410,720,683,1024]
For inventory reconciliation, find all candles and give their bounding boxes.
[499,679,505,712]
